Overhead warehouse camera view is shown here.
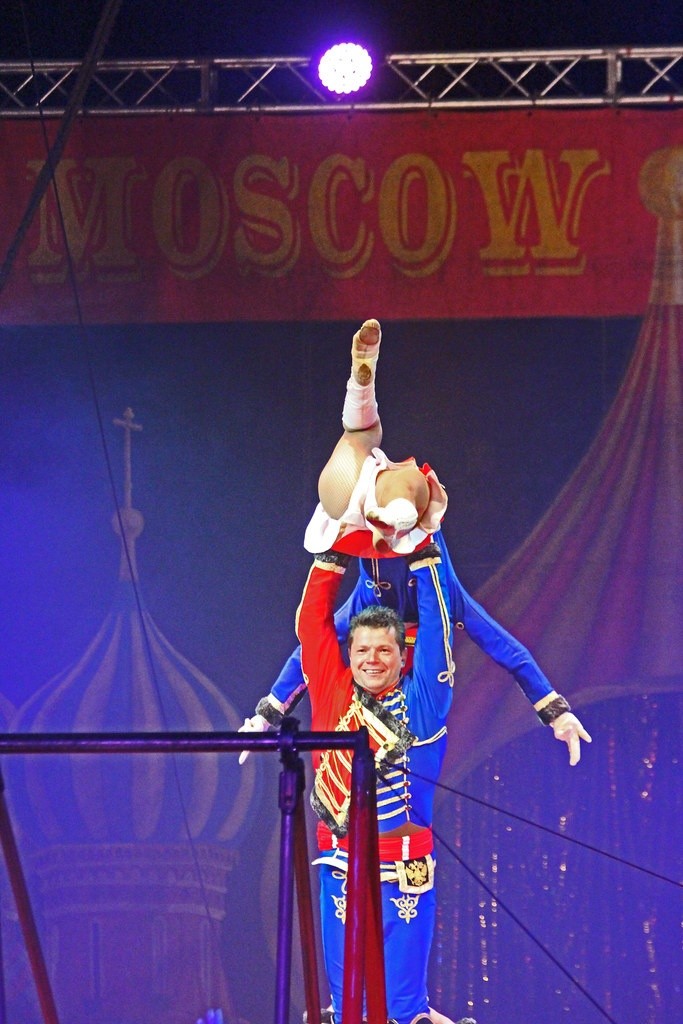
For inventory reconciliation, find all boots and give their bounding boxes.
[343,318,382,430]
[366,498,418,554]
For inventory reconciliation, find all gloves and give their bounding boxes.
[550,712,592,768]
[237,712,270,765]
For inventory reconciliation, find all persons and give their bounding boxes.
[235,315,595,768]
[296,542,457,1024]
